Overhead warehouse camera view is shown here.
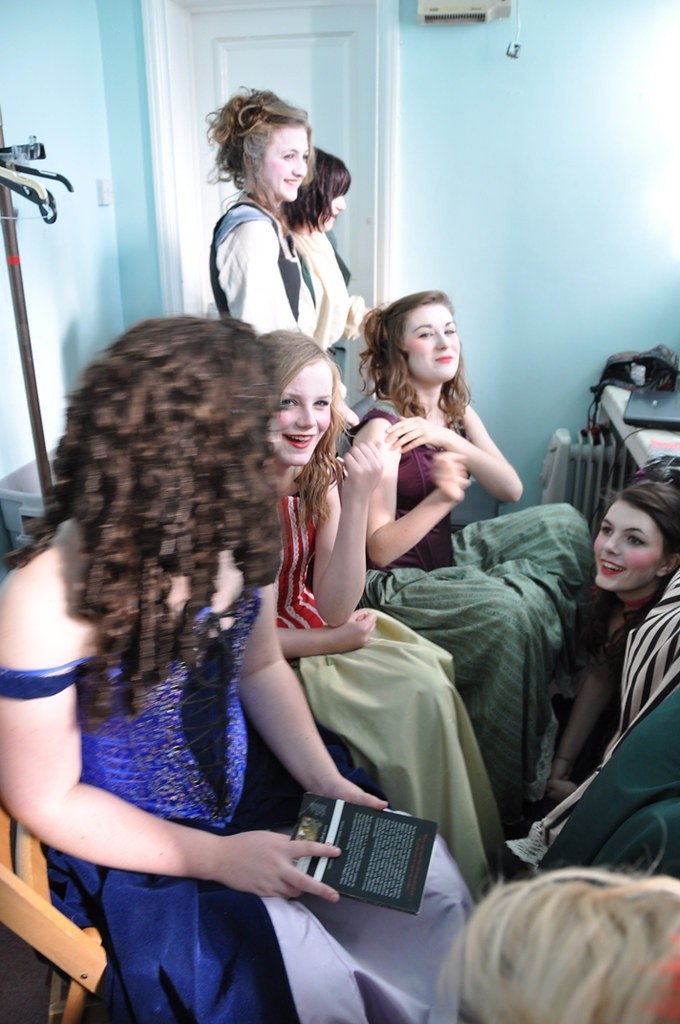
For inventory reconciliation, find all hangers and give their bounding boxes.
[0,135,73,224]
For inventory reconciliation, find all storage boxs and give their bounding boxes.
[0,445,61,552]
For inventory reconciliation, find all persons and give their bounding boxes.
[0,86,680,1024]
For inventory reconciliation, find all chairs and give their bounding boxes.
[0,806,108,1024]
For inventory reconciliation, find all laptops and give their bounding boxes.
[623,388,680,430]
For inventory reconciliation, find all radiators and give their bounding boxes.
[540,428,640,544]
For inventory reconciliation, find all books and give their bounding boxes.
[289,791,438,916]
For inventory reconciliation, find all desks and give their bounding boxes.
[600,386,680,469]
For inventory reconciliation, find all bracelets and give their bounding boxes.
[554,749,575,764]
